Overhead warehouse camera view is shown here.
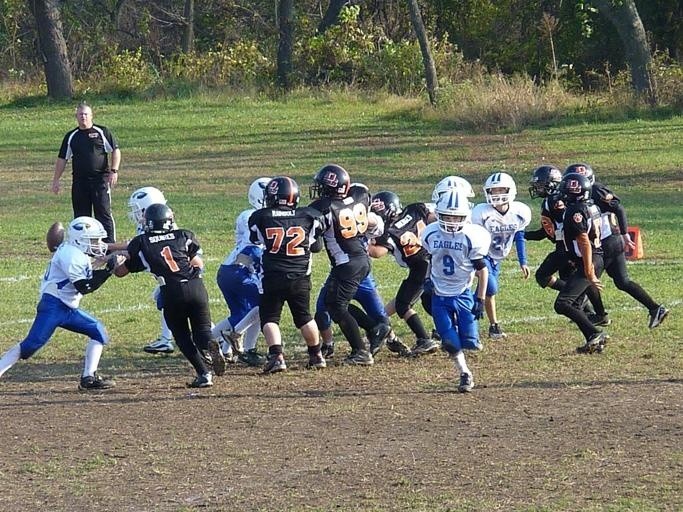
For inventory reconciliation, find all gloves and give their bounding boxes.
[472,296,484,320]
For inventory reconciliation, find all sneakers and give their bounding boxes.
[576,311,611,354]
[144,338,174,354]
[488,323,507,339]
[459,373,475,392]
[190,341,287,387]
[78,372,116,391]
[648,304,669,329]
[303,324,442,369]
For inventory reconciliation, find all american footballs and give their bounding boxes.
[47,222,65,252]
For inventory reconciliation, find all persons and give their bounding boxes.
[0,215,128,391]
[51,104,122,258]
[90,161,670,393]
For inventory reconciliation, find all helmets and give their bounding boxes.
[128,186,173,234]
[67,216,108,258]
[248,164,404,217]
[431,164,595,233]
